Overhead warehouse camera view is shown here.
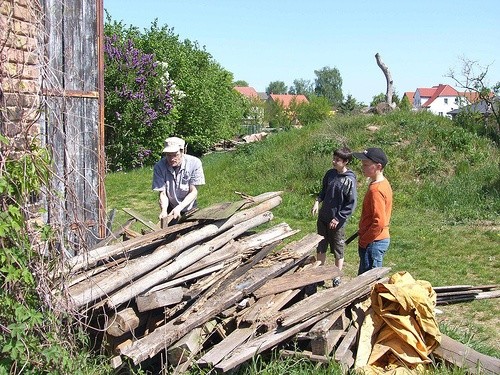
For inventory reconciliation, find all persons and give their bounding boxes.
[350,147,393,275]
[152,137,205,220]
[311,146,359,286]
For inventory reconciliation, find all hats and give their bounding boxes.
[353,148,387,168]
[162,137,185,152]
